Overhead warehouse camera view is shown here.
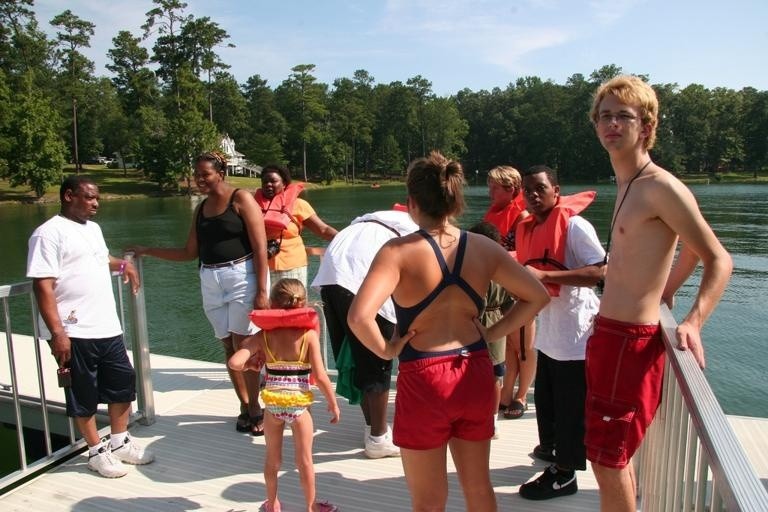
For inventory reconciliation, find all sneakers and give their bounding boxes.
[518,464,578,501]
[363,425,402,459]
[363,424,372,446]
[533,444,558,462]
[108,430,156,465]
[87,438,129,479]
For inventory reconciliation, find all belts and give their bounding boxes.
[202,253,254,269]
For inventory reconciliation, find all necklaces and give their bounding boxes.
[603,160,650,269]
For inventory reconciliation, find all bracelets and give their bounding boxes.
[119,259,126,276]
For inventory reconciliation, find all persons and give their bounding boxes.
[126,152,270,437]
[311,210,419,460]
[467,222,515,439]
[509,165,604,501]
[585,74,733,509]
[227,278,340,512]
[25,177,154,478]
[486,165,537,421]
[254,165,337,288]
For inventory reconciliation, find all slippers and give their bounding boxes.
[316,500,339,512]
[250,408,267,436]
[503,399,530,419]
[261,499,284,512]
[499,403,507,410]
[235,412,252,433]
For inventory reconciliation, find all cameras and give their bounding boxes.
[267,240,279,258]
